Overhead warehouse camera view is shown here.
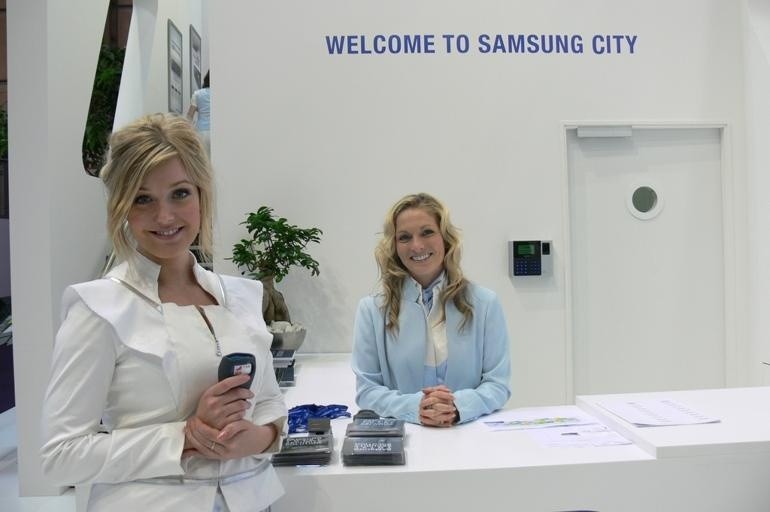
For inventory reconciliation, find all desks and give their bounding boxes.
[267,352,656,512]
[575,385,770,512]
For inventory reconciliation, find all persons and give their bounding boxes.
[35,112,291,512]
[346,189,514,431]
[182,68,210,157]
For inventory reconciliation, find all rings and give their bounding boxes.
[209,441,217,451]
[440,420,443,424]
[425,404,431,409]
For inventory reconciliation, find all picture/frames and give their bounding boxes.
[188,23,203,102]
[168,18,183,115]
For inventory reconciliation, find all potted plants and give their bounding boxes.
[225,202,323,350]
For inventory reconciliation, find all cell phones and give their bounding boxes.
[218,352,256,401]
